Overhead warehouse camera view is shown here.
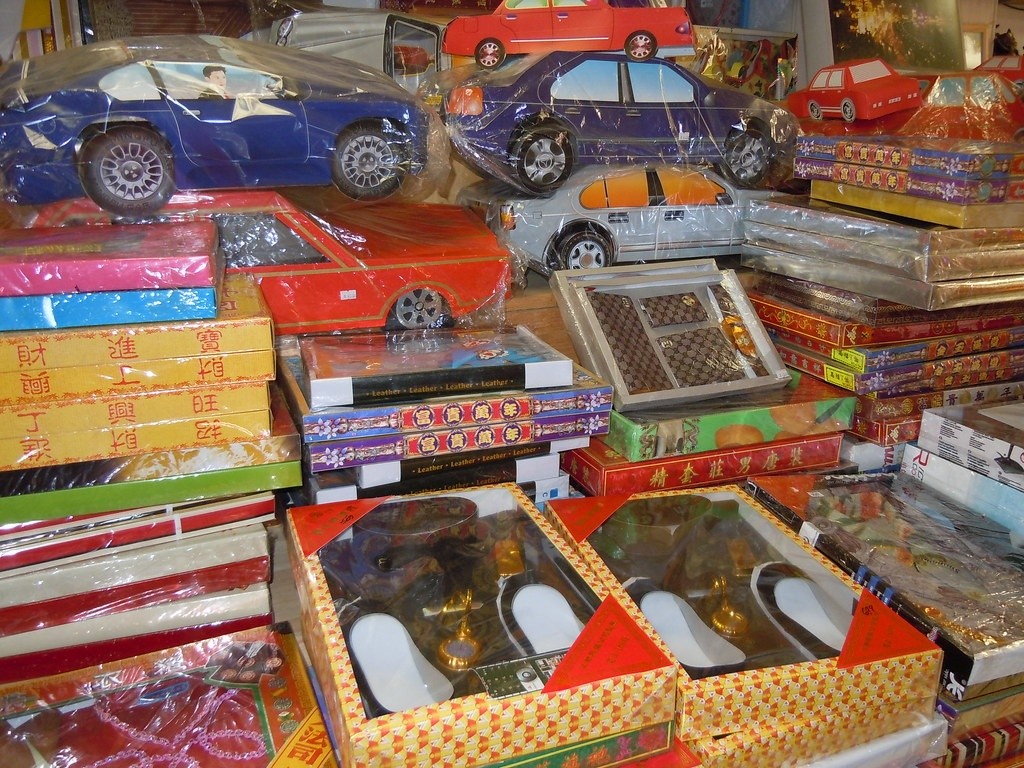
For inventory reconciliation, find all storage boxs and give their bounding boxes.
[0,24,1024,768]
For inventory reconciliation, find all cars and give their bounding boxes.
[440,50,800,193]
[459,163,791,280]
[785,58,924,121]
[262,13,483,93]
[13,194,507,334]
[0,37,432,220]
[440,0,696,71]
